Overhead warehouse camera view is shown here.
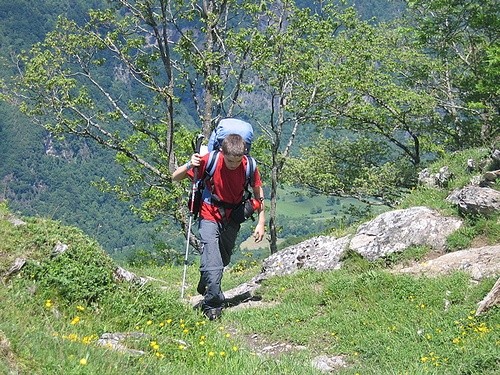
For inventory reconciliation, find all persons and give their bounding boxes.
[171,133,265,321]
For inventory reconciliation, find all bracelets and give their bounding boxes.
[186,161,192,170]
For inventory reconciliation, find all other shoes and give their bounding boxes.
[202,305,220,323]
[197,278,207,293]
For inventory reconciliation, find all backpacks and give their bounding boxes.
[206,118,257,185]
